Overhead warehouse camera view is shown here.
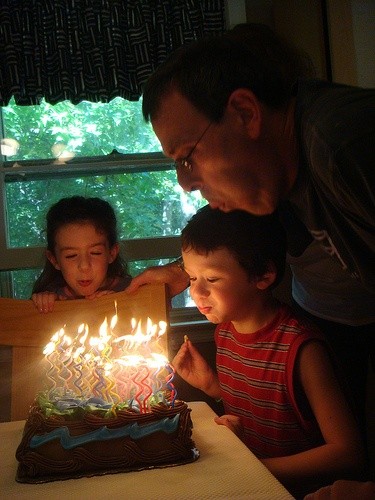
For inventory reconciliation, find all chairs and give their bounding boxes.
[0,280,176,424]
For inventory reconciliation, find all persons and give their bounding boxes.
[26,197,134,315]
[173,202,366,500]
[121,28,375,500]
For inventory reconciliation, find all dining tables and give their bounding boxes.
[0,400,297,500]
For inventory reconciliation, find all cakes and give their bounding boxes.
[12,301,201,485]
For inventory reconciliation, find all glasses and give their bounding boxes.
[174,118,216,170]
